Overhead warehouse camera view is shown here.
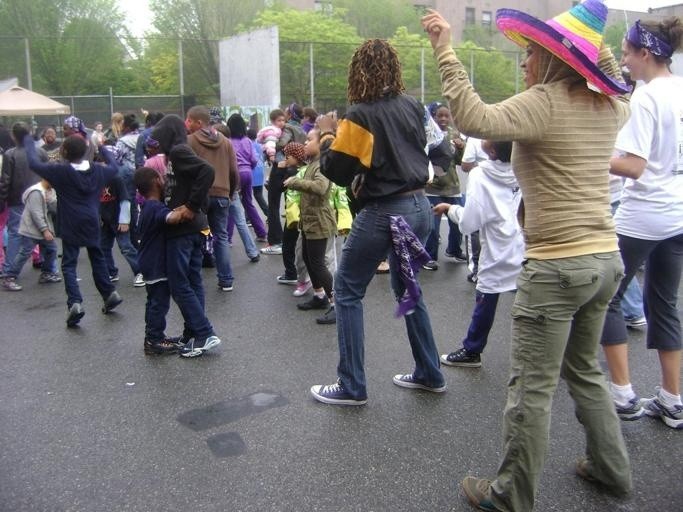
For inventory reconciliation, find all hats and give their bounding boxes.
[495,0,634,96]
[282,142,307,163]
[209,106,222,123]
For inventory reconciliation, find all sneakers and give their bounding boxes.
[575,456,633,498]
[463,476,501,512]
[229,210,286,262]
[216,284,233,291]
[392,373,447,392]
[640,386,683,429]
[310,377,368,406]
[66,303,84,328]
[264,182,269,190]
[202,254,216,267]
[109,274,119,283]
[441,348,482,368]
[145,334,221,358]
[277,274,298,285]
[444,247,466,262]
[376,261,390,273]
[0,270,23,291]
[102,290,123,313]
[422,260,438,270]
[133,273,146,287]
[33,259,62,283]
[626,316,648,327]
[293,281,335,324]
[575,381,645,425]
